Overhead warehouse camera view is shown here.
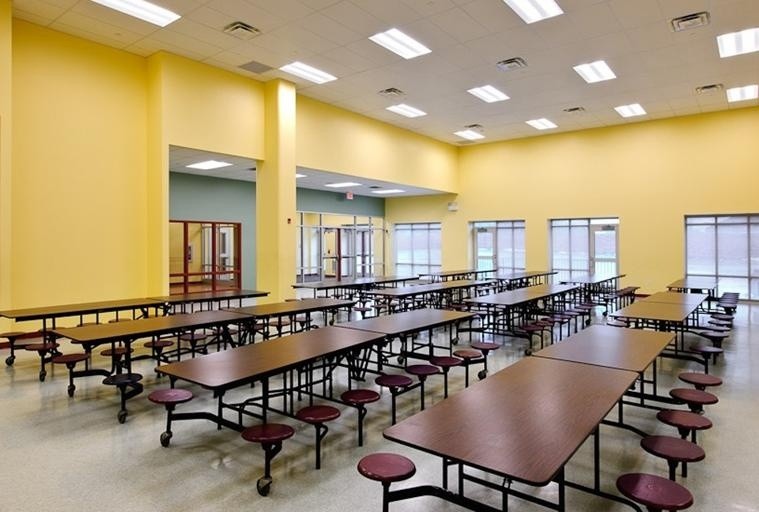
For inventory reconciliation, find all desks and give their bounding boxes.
[1,288,270,328]
[147,307,477,408]
[48,294,359,361]
[293,265,629,306]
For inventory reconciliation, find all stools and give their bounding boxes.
[453,347,483,389]
[427,355,462,400]
[405,363,441,414]
[469,339,501,375]
[522,284,643,351]
[372,371,414,425]
[354,449,417,512]
[616,470,694,512]
[295,402,341,472]
[679,371,724,391]
[2,329,188,440]
[239,420,296,500]
[640,433,707,484]
[668,385,720,444]
[654,409,714,477]
[698,287,741,373]
[338,387,385,448]
[282,275,531,331]
